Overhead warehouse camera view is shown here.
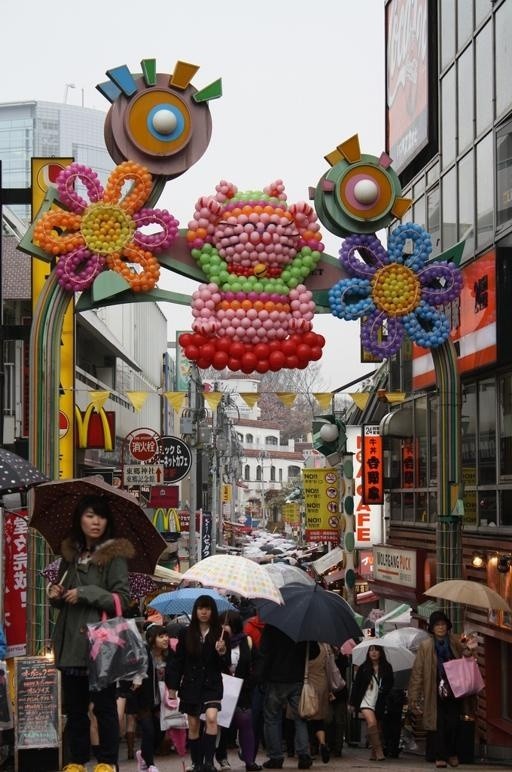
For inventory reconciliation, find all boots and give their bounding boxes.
[367,725,387,761]
[124,730,135,761]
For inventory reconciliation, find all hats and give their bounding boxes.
[426,610,454,633]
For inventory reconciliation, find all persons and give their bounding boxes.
[47,498,137,772]
[88,594,474,772]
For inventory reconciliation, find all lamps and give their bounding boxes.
[495,553,511,574]
[471,549,486,567]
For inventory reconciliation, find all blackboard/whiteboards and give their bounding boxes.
[14,656,62,748]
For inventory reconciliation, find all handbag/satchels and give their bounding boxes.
[297,682,325,722]
[158,680,189,732]
[320,642,347,693]
[85,592,150,692]
[374,695,393,720]
[438,657,487,708]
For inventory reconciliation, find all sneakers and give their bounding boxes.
[434,759,448,768]
[94,761,115,772]
[446,755,460,766]
[145,766,161,772]
[61,761,87,772]
[174,742,342,771]
[135,749,148,772]
